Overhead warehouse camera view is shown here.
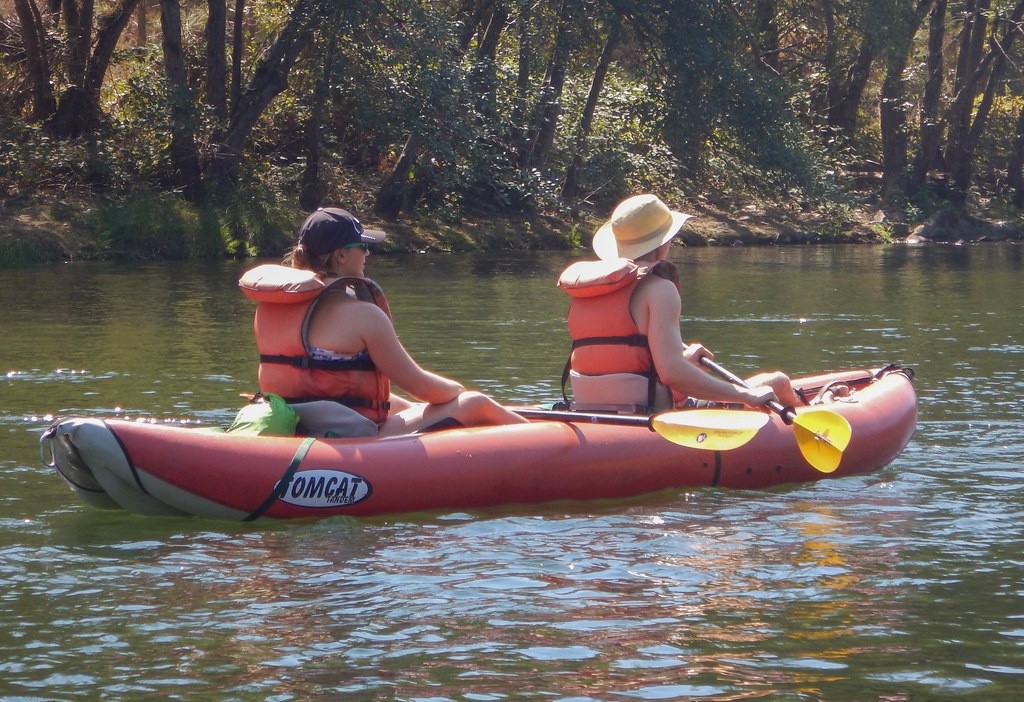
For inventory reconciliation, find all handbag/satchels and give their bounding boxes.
[226,392,300,436]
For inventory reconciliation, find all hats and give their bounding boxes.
[298,207,387,259]
[592,194,698,261]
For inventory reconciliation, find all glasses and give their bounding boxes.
[341,242,368,254]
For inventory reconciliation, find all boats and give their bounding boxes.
[39,365,918,525]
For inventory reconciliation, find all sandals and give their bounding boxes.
[808,380,850,406]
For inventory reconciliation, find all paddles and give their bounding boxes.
[679,342,853,472]
[239,392,769,450]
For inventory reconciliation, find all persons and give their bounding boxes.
[237,206,531,439]
[555,194,851,414]
